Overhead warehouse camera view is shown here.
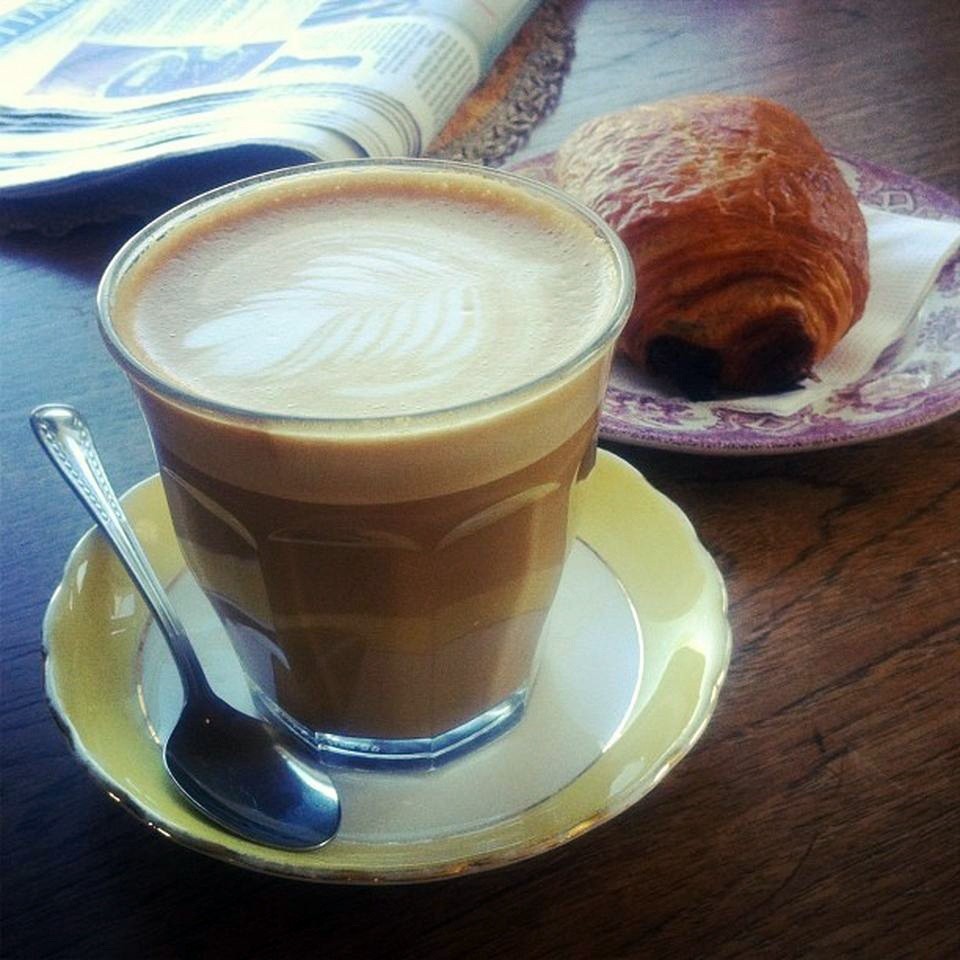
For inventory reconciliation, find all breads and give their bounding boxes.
[551,91,873,393]
